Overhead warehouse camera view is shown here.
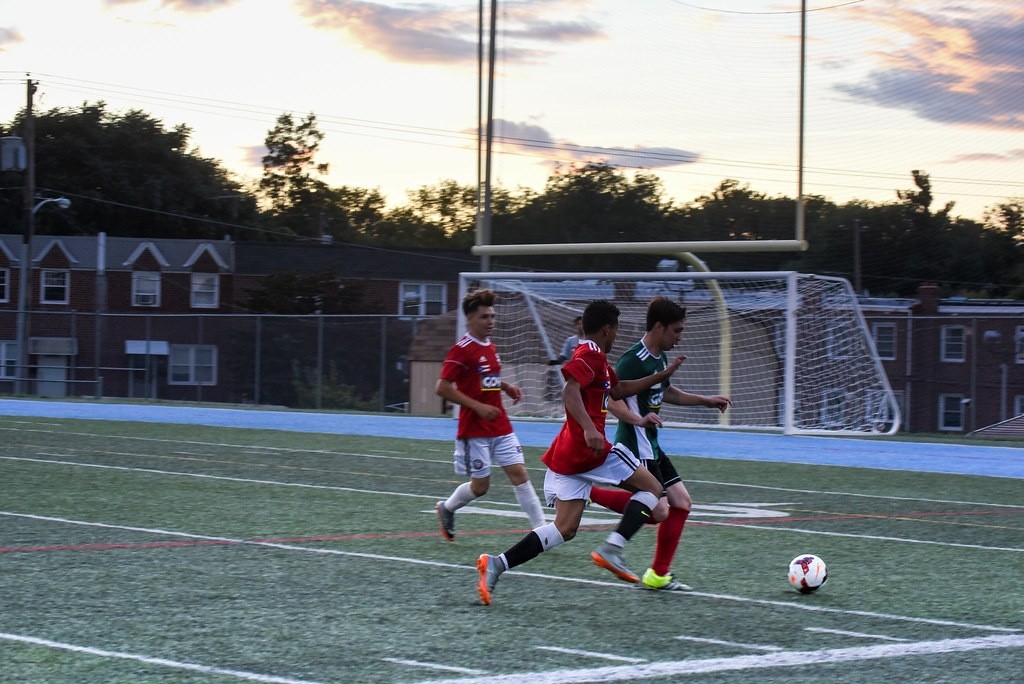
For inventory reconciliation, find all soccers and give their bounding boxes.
[787,553,830,594]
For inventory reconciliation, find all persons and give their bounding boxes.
[435,288,550,540]
[547,316,584,366]
[477,300,663,606]
[585,297,733,591]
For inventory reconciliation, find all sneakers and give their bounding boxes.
[642,568,695,590]
[590,541,641,584]
[476,554,502,606]
[585,497,593,508]
[434,501,456,541]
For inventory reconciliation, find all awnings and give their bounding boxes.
[125,339,170,355]
